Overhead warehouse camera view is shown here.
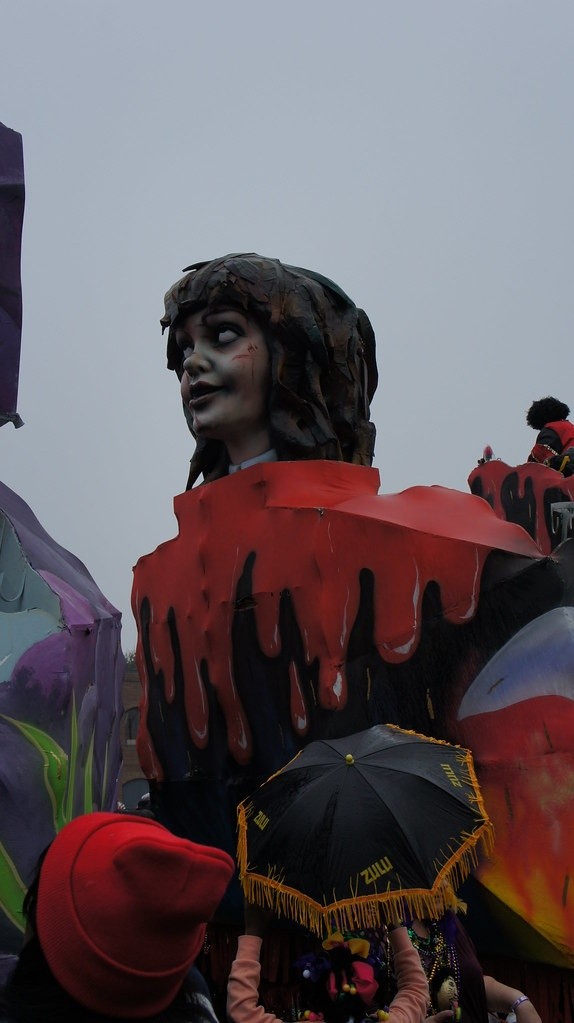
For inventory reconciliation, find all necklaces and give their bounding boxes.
[381,920,460,1014]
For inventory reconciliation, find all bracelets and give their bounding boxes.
[511,996,528,1010]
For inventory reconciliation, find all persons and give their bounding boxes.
[160,254,379,488]
[0,812,234,1023]
[526,398,574,476]
[303,907,542,1023]
[225,898,430,1023]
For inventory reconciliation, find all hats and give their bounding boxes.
[35,812,236,1019]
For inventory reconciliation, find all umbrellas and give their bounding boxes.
[236,724,495,933]
